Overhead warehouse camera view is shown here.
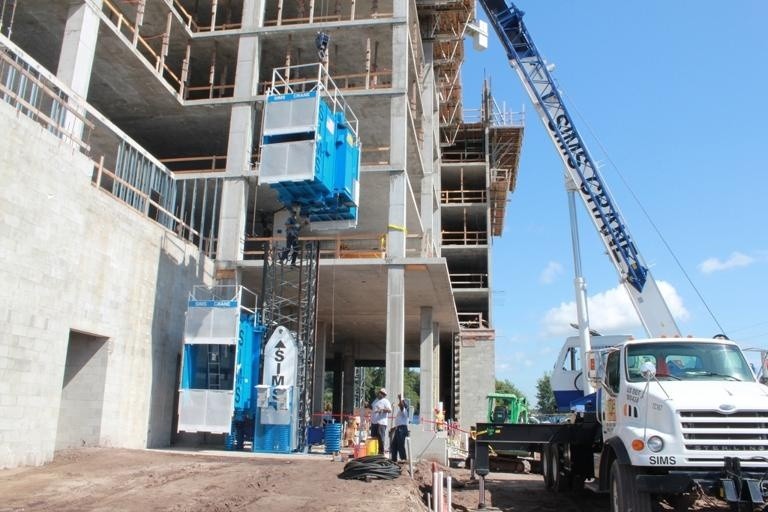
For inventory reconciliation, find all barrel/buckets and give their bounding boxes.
[366,437,379,456]
[324,423,341,454]
[354,444,366,459]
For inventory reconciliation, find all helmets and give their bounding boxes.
[404,400,409,407]
[379,389,386,395]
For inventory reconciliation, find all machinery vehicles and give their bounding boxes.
[462,390,541,474]
[478,0,768,511]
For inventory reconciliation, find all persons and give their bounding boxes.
[323,404,334,425]
[280,209,310,269]
[435,408,443,430]
[501,399,509,421]
[370,389,393,455]
[389,393,411,463]
